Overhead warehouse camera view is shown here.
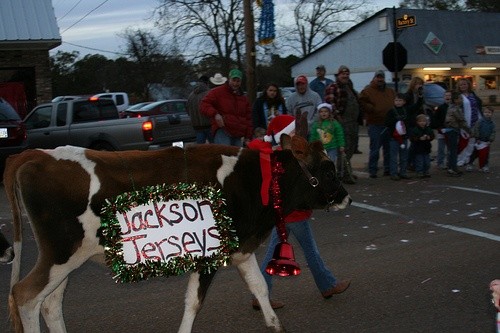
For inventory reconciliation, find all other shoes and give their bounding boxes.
[353,149,362,154]
[384,170,400,176]
[397,155,474,178]
[479,167,490,172]
[322,279,352,299]
[391,175,402,181]
[370,175,377,178]
[252,294,286,310]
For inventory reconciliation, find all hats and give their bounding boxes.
[198,75,210,82]
[338,65,350,75]
[317,65,325,69]
[264,114,296,145]
[317,102,333,112]
[374,70,386,79]
[393,130,405,149]
[230,69,242,80]
[210,72,227,85]
[296,76,307,85]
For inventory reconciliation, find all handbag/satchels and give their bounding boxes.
[396,120,406,136]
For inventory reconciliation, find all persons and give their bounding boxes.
[189,64,496,181]
[251,115,352,312]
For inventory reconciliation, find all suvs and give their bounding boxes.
[0,96,26,154]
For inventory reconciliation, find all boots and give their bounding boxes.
[336,158,358,185]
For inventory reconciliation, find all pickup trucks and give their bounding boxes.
[22,97,154,152]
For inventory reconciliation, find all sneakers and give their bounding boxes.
[0,246,15,265]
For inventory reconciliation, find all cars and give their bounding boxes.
[52,91,195,144]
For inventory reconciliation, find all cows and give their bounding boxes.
[2,105,352,333]
